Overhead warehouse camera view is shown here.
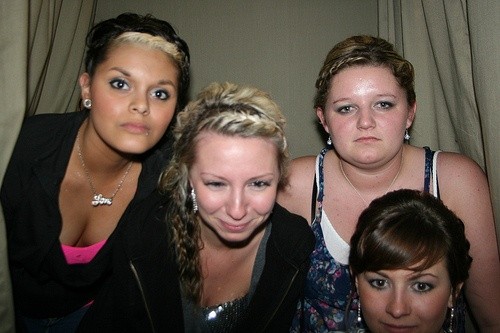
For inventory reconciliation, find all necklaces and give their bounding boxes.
[77,136,132,206]
[339,156,403,205]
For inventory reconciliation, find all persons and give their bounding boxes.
[0,13,191,333]
[348,189,471,333]
[276,35,500,333]
[76,104,316,333]
